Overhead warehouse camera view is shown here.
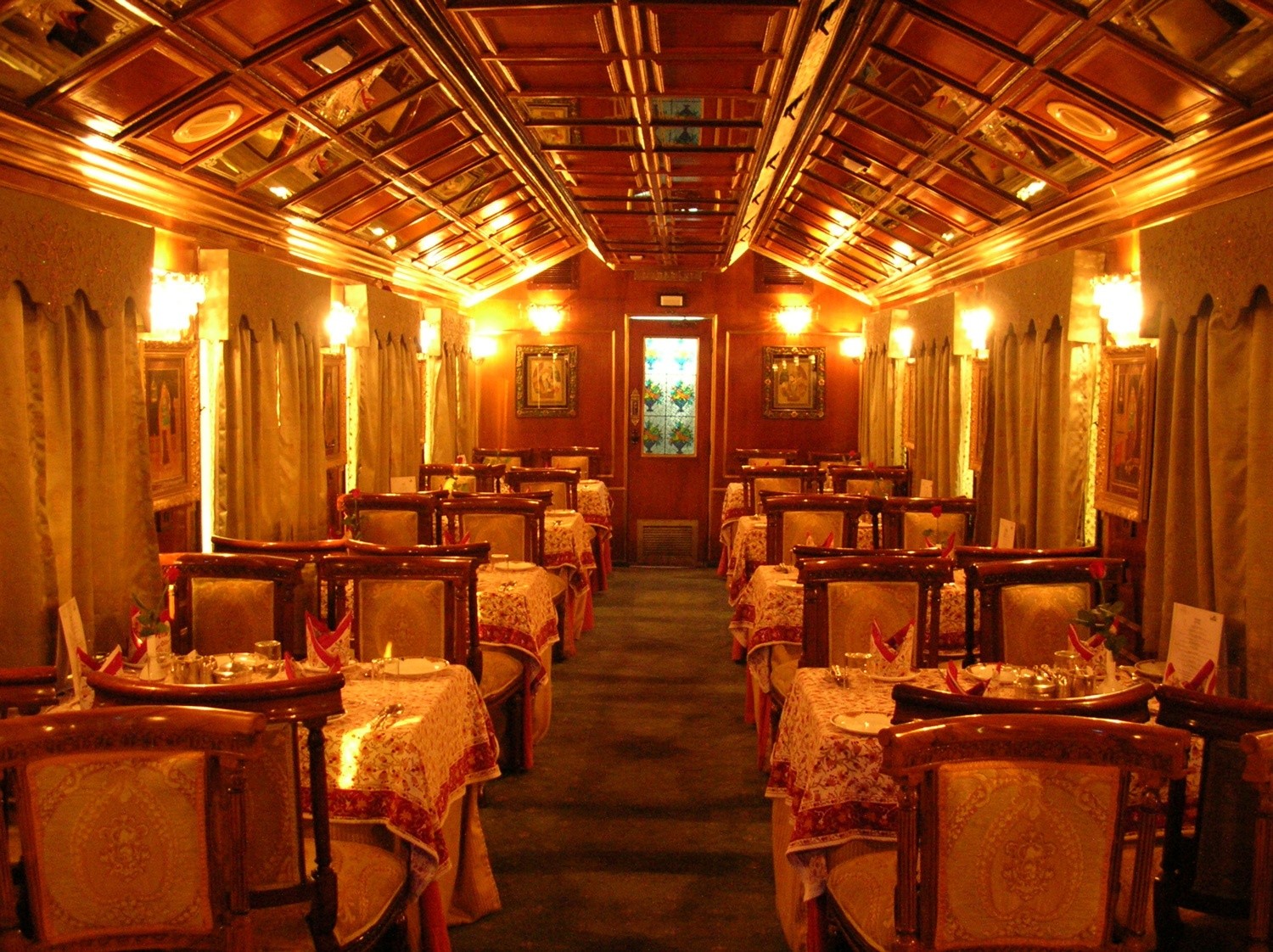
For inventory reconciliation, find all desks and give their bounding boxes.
[728,565,980,775]
[432,513,597,640]
[320,566,560,770]
[726,515,881,607]
[494,477,614,590]
[765,666,1204,952]
[35,647,501,952]
[716,482,834,580]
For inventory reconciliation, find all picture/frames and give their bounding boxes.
[1093,344,1157,522]
[321,344,348,470]
[137,339,201,512]
[516,345,578,418]
[762,346,826,420]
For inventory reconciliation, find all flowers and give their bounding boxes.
[922,506,949,548]
[131,564,181,637]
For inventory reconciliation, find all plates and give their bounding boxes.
[547,509,578,514]
[776,580,805,588]
[580,480,599,484]
[382,654,451,676]
[830,712,916,737]
[1136,659,1167,678]
[754,523,770,528]
[861,665,923,681]
[496,561,535,570]
[965,662,1023,684]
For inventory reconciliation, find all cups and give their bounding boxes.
[842,652,876,710]
[147,639,283,684]
[1015,650,1096,698]
[371,658,401,709]
[492,554,510,590]
[1216,666,1240,698]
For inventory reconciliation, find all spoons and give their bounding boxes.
[375,704,405,729]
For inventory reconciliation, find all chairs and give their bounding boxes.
[0,445,602,952]
[724,448,1273,952]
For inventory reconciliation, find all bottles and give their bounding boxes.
[212,651,253,685]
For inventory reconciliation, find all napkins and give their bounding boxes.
[74,645,125,709]
[305,609,352,668]
[1067,623,1110,675]
[870,619,916,677]
